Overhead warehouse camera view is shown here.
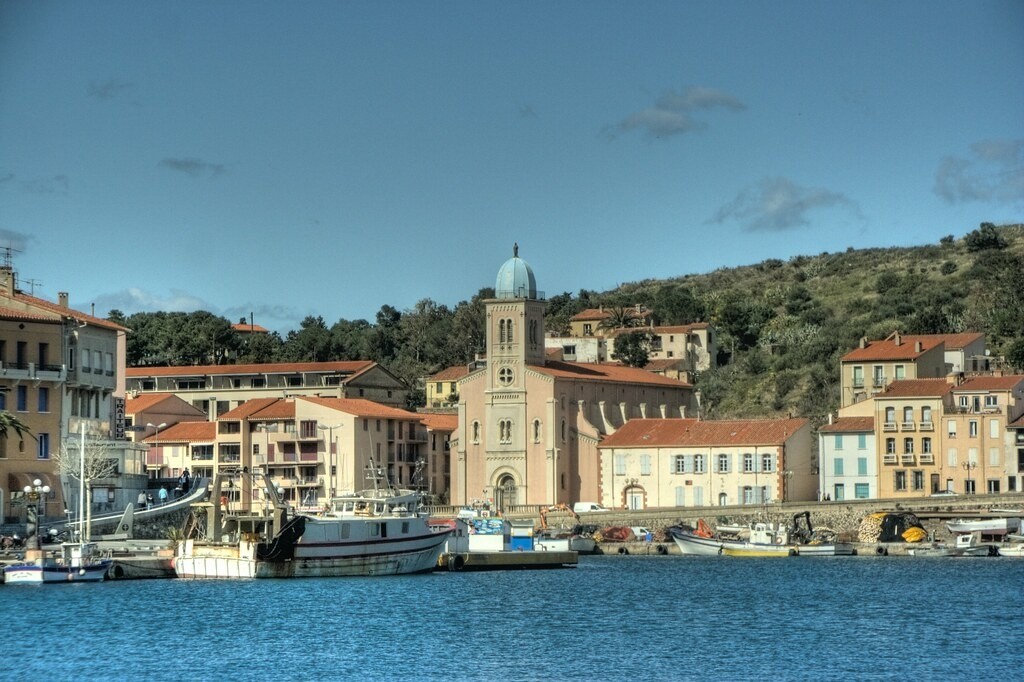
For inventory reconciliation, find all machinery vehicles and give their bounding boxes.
[537,503,582,537]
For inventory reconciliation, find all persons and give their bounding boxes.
[158,485,170,506]
[136,489,147,511]
[147,494,154,511]
[175,467,191,492]
[173,484,185,501]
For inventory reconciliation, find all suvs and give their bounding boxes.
[574,501,611,513]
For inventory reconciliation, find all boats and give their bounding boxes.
[0,461,458,586]
[533,519,1024,559]
